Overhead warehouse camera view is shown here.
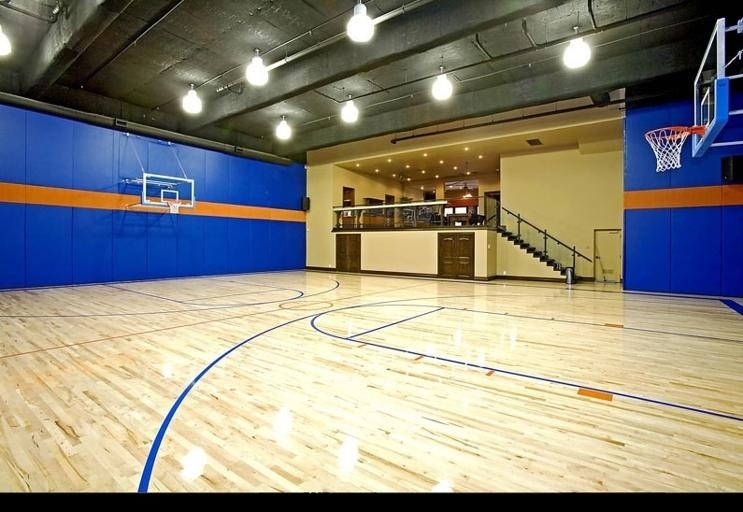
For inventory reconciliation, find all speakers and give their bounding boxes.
[720,155,743,182]
[302,196,310,211]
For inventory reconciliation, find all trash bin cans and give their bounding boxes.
[565,267,574,284]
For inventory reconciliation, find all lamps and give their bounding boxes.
[275,115,292,141]
[179,0,376,118]
[564,26,591,68]
[0,24,13,62]
[341,95,358,123]
[431,65,453,100]
